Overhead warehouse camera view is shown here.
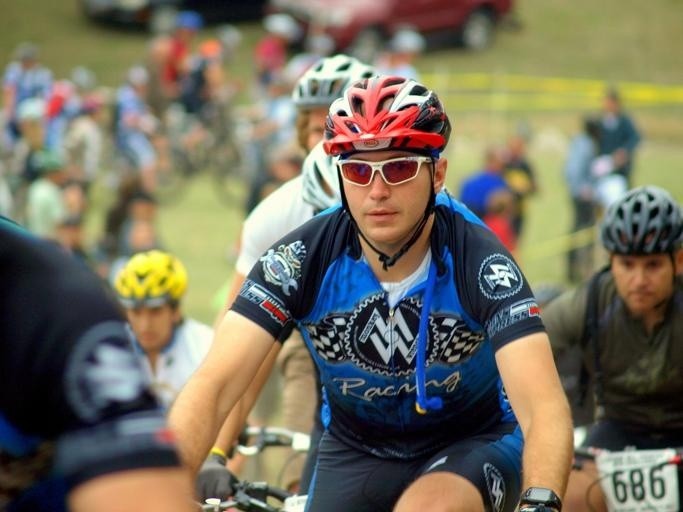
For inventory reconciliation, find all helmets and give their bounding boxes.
[292,52,375,107]
[600,184,683,257]
[115,249,188,310]
[323,73,452,159]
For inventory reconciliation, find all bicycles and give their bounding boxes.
[199,420,683,512]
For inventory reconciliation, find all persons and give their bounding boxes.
[541,185,683,512]
[196,55,377,500]
[566,93,638,283]
[117,251,214,404]
[1,12,232,276]
[1,215,202,512]
[247,15,424,213]
[456,137,534,248]
[169,77,573,511]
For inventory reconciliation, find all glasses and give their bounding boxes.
[335,156,433,188]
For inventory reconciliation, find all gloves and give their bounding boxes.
[194,446,239,506]
